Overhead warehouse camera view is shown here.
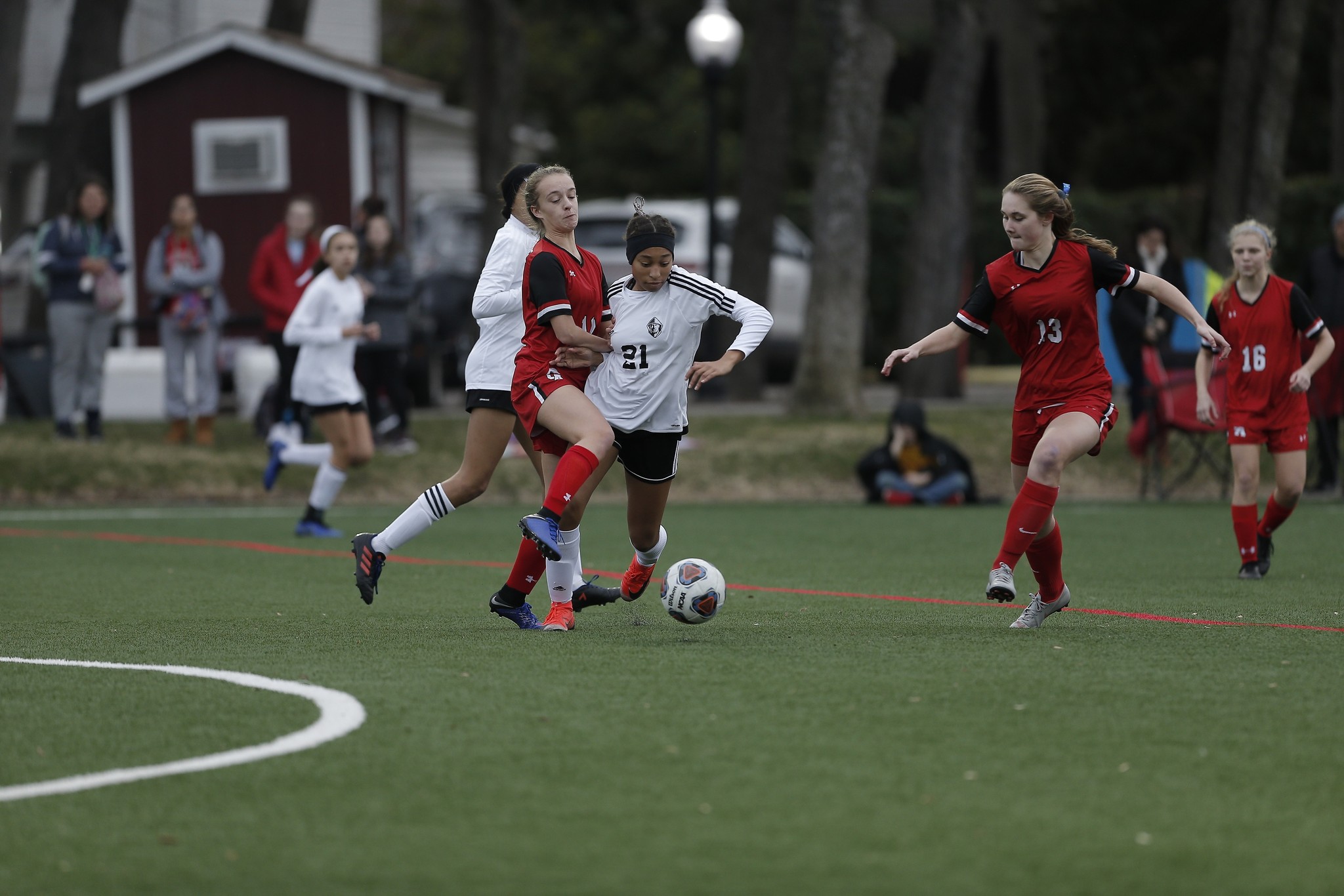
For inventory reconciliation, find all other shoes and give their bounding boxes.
[195,417,216,444]
[85,412,104,442]
[296,519,345,538]
[56,422,76,439]
[264,442,286,490]
[167,420,188,444]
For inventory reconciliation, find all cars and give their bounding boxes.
[353,269,482,408]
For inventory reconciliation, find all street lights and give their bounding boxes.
[686,1,745,403]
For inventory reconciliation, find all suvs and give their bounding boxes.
[573,194,817,385]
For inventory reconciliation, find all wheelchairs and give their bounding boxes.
[1127,328,1235,507]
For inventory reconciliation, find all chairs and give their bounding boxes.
[1141,343,1232,500]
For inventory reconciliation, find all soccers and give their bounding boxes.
[661,558,726,626]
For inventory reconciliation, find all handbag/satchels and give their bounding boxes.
[95,266,123,312]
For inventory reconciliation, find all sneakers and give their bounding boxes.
[985,562,1017,603]
[542,600,575,632]
[1238,561,1262,580]
[620,525,667,601]
[489,590,543,630]
[1010,583,1071,628]
[351,532,386,605]
[517,513,566,561]
[570,574,620,612]
[1254,520,1273,574]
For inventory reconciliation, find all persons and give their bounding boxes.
[1107,213,1189,424]
[351,196,421,454]
[0,177,127,438]
[857,403,975,507]
[880,173,1232,628]
[248,192,326,440]
[142,194,230,446]
[544,214,774,631]
[1195,220,1336,578]
[351,162,621,613]
[262,223,382,538]
[488,167,615,603]
[1299,203,1344,489]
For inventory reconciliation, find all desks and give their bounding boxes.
[94,346,193,420]
